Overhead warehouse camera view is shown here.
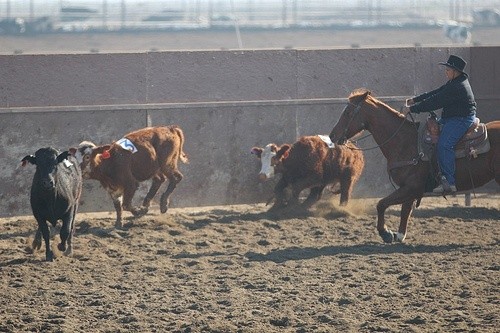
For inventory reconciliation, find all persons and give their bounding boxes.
[403,54,477,193]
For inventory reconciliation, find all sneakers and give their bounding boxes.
[433,183,456,193]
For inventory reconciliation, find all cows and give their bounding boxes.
[21,146,83,261]
[250,134,365,210]
[78,124,190,228]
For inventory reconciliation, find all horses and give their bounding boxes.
[329,87,500,244]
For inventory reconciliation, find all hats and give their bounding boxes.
[438,54,470,77]
